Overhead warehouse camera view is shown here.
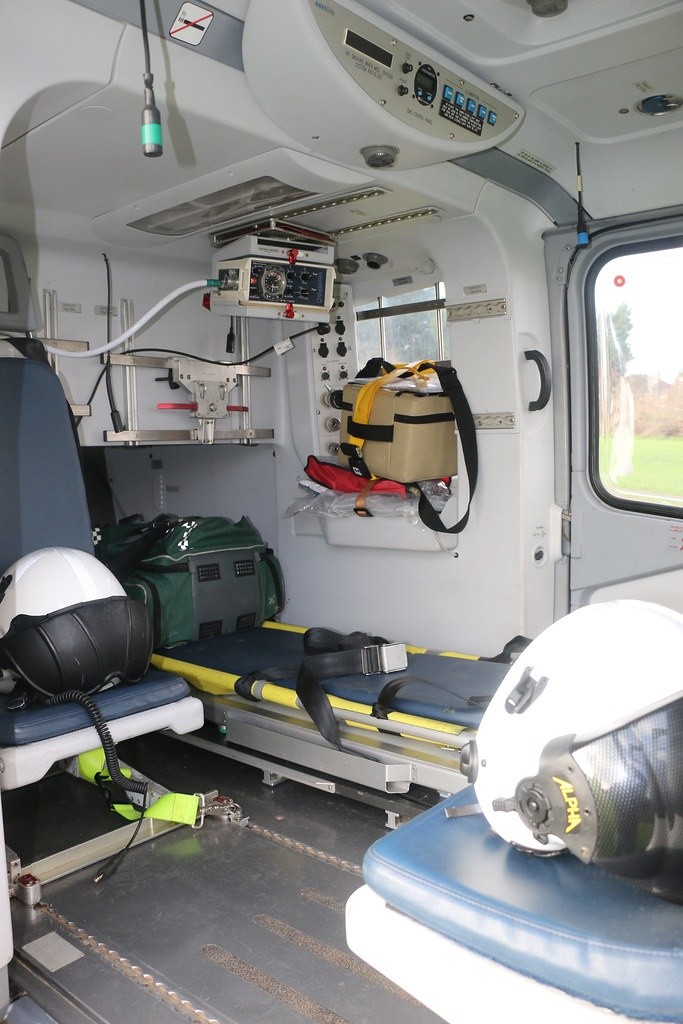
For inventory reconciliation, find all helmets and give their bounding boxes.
[458,599,683,858]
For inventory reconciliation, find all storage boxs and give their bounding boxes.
[338,381,459,483]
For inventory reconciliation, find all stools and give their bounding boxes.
[345,783,683,1024]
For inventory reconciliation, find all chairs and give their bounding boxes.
[0,356,204,789]
